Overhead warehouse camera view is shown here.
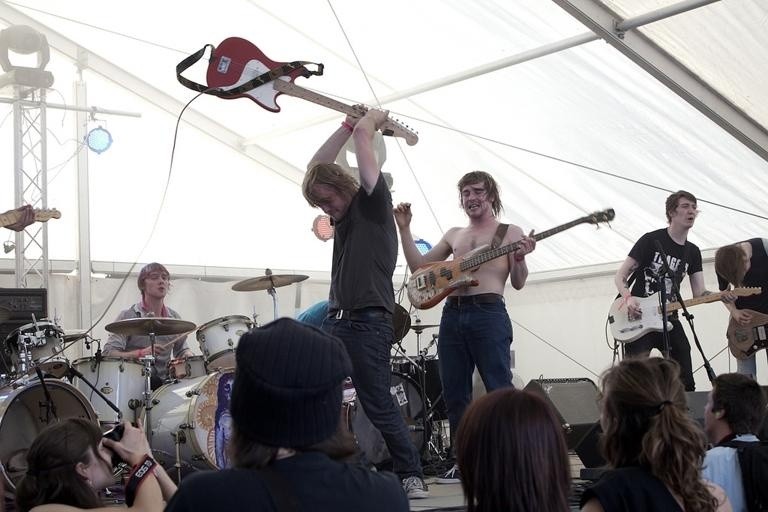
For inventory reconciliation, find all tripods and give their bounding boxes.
[416,339,452,473]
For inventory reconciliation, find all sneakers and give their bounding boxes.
[437,466,461,484]
[404,475,430,499]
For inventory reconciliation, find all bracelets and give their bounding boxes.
[138,349,143,358]
[341,119,351,131]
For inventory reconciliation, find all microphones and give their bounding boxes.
[85,341,90,350]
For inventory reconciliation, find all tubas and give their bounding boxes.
[608,286,762,343]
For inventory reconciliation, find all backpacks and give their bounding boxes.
[718,439,768,512]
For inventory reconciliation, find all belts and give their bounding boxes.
[333,309,386,320]
[446,293,503,306]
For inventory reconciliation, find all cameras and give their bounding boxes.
[103,421,139,466]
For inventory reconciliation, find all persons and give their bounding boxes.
[616,191,739,392]
[457,387,573,512]
[163,318,411,512]
[13,417,178,511]
[297,104,430,498]
[698,375,768,512]
[395,173,536,482]
[579,356,734,511]
[715,238,768,382]
[103,263,195,388]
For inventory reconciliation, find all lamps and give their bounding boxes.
[414,239,432,255]
[311,215,335,242]
[87,126,112,154]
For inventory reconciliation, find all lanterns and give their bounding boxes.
[622,294,631,300]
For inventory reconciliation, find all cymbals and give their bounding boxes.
[105,317,197,336]
[394,304,411,344]
[63,334,87,342]
[230,275,309,290]
[412,324,438,329]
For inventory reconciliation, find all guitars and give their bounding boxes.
[727,309,768,360]
[206,37,418,145]
[407,208,615,310]
[0,207,61,227]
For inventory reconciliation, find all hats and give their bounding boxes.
[235,317,353,447]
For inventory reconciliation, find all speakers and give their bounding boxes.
[522,378,603,451]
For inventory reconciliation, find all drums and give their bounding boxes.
[3,322,69,380]
[196,315,252,373]
[169,355,208,380]
[141,367,236,471]
[73,357,151,426]
[393,359,448,420]
[0,379,100,494]
[340,374,433,472]
